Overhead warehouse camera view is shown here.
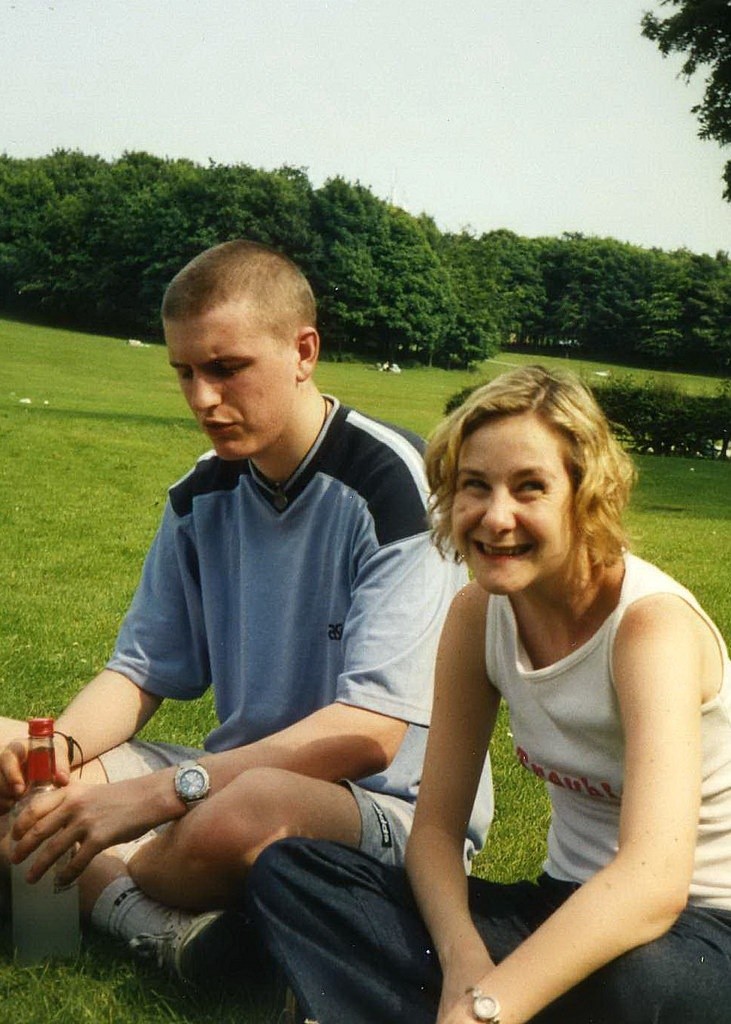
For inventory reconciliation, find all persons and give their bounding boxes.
[0,240,496,986]
[245,363,731,1024]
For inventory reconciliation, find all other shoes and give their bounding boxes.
[128,909,239,990]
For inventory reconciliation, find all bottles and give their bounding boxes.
[8,718,84,967]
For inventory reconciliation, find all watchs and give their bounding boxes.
[174,759,212,811]
[465,986,503,1024]
